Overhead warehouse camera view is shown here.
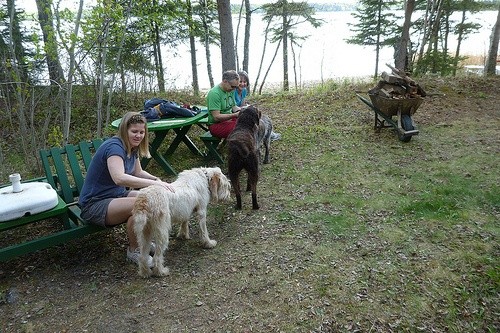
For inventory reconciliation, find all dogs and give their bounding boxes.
[227,104,273,211]
[132,167,231,278]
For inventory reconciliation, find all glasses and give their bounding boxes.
[228,81,237,88]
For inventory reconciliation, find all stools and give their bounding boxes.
[192,118,210,131]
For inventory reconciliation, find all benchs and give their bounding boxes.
[200,130,226,164]
[39,136,127,235]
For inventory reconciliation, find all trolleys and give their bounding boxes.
[356,63,424,143]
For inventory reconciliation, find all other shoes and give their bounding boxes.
[270,131,281,142]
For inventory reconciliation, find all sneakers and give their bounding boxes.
[126,245,154,268]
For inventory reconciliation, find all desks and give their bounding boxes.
[111,104,208,174]
[0,172,79,260]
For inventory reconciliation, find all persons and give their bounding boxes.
[77,111,176,268]
[233,70,281,144]
[205,69,250,140]
